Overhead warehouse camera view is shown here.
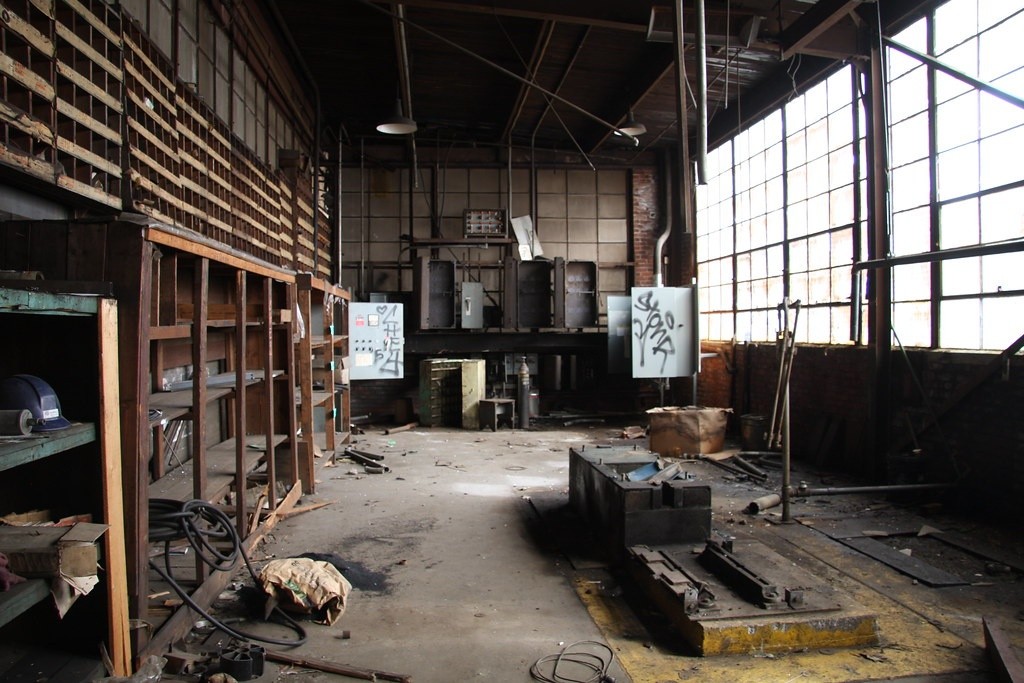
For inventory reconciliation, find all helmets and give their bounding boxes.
[0,374,71,431]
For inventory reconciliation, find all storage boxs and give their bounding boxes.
[645,406,733,457]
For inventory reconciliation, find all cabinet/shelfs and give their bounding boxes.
[418,358,487,431]
[4,288,131,683]
[418,257,456,330]
[296,269,354,493]
[120,225,302,674]
